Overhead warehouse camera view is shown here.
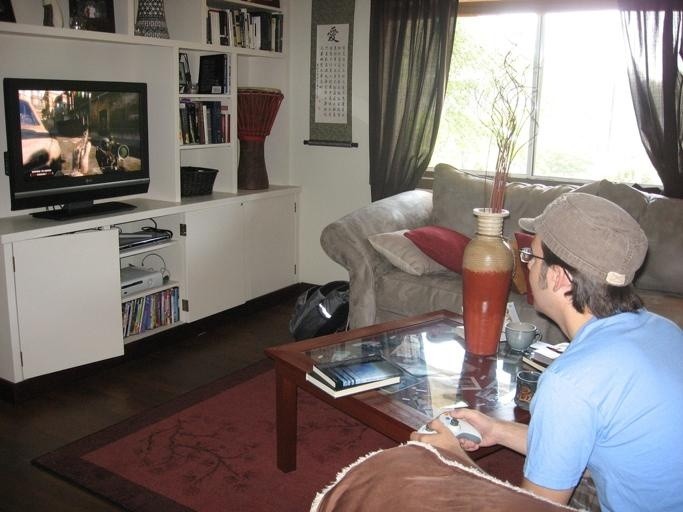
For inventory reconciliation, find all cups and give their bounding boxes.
[513,371,538,411]
[505,320,544,351]
[70,16,84,30]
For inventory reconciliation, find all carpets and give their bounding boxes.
[29,357,527,512]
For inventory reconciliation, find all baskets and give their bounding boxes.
[181,166,218,195]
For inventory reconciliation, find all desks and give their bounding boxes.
[262,309,550,473]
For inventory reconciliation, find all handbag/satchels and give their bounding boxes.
[291,282,351,341]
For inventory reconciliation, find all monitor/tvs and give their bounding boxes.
[2,77,150,222]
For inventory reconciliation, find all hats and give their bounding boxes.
[519,192,649,287]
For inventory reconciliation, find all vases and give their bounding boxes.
[461,209,515,356]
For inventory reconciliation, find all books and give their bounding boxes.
[525,338,578,366]
[522,349,549,375]
[177,1,288,144]
[313,354,407,389]
[453,301,523,343]
[305,364,402,399]
[120,287,182,340]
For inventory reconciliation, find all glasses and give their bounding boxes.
[519,247,546,263]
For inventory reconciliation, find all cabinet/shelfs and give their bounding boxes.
[0,0,302,406]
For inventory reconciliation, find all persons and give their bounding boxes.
[407,188,683,512]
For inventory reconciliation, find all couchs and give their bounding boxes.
[320,187,683,345]
